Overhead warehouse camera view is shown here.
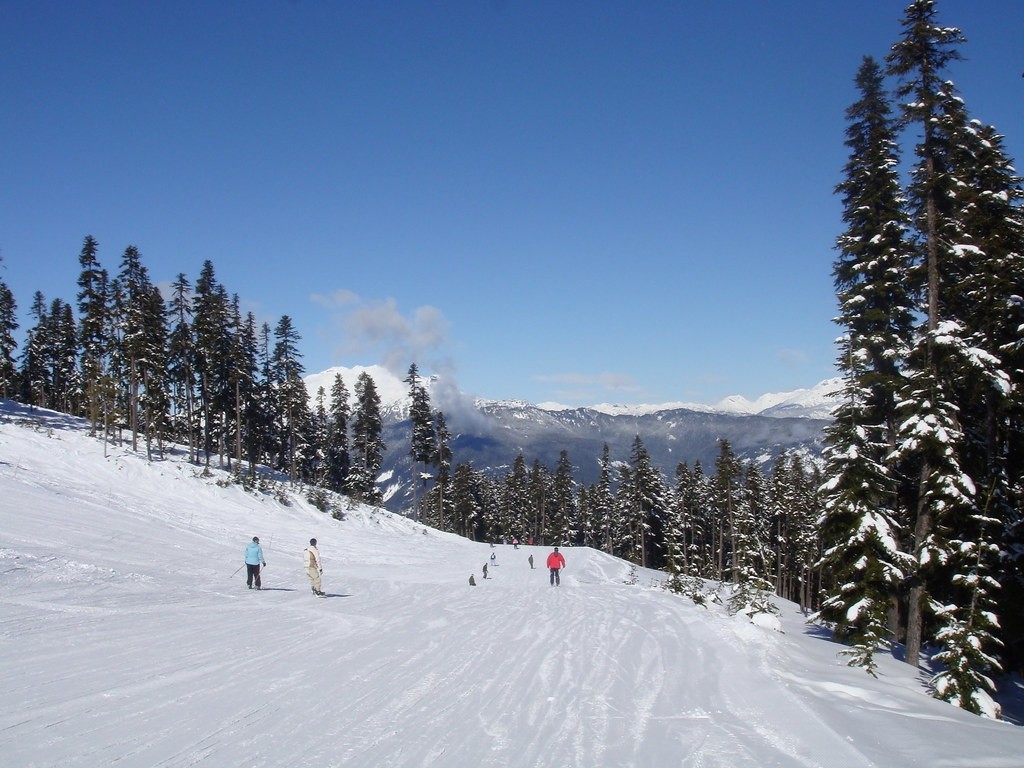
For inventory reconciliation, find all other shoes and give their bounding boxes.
[316,591,324,595]
[249,585,252,589]
[255,585,260,590]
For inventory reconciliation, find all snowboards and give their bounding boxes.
[310,585,328,599]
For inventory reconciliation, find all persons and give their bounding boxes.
[491,553,496,566]
[244,536,266,590]
[468,574,477,586]
[501,533,518,549]
[490,536,496,547]
[483,563,488,579]
[304,538,326,598]
[547,547,565,586]
[528,555,533,569]
